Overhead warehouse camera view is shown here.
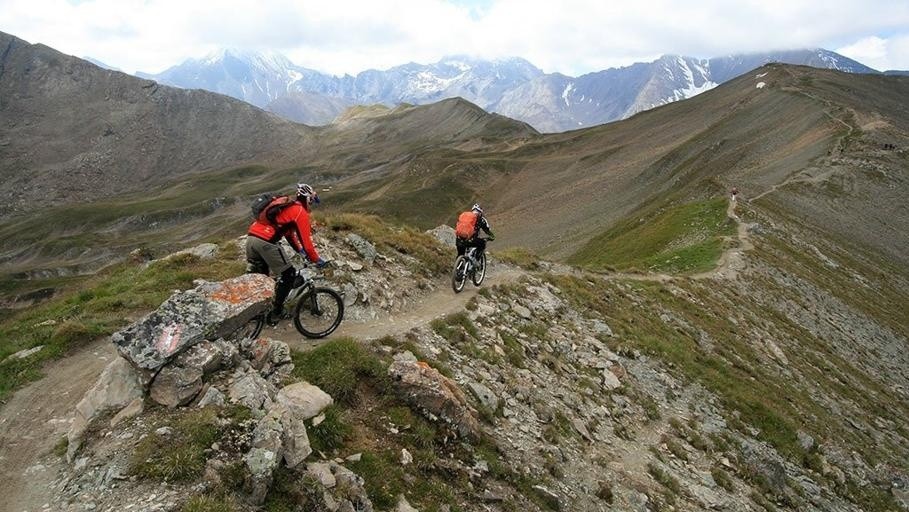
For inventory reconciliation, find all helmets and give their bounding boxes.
[294,182,320,213]
[471,203,484,216]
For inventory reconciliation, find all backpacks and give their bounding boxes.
[249,191,305,230]
[455,211,482,243]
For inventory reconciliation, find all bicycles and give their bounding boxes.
[452,237,494,293]
[224,256,344,341]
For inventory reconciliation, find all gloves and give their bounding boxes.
[298,248,331,268]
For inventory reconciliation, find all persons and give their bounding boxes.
[453,203,495,280]
[242,182,328,326]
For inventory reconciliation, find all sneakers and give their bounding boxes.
[270,310,295,324]
[473,259,482,268]
